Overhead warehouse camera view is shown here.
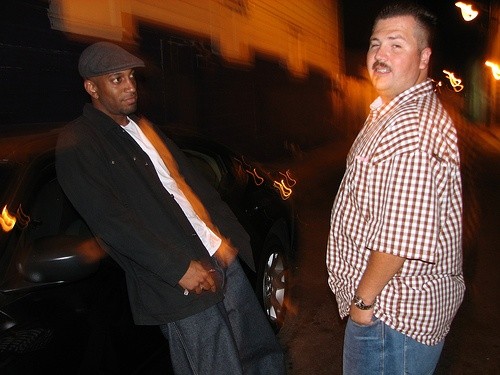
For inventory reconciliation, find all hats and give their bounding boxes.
[78,42,146,78]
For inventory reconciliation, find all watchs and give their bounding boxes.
[351,295,375,311]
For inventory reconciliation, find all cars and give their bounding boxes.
[0,129,302,375]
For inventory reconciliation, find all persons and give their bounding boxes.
[54,41,288,375]
[326,11,466,375]
